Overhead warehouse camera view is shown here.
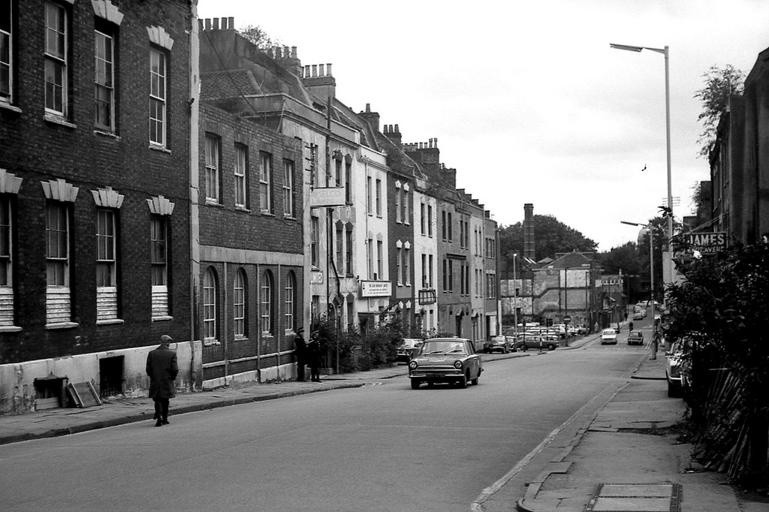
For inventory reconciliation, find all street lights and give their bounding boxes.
[609,42,676,287]
[620,221,656,360]
[585,271,591,336]
[565,266,570,346]
[513,253,518,349]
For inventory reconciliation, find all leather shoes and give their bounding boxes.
[155,417,170,426]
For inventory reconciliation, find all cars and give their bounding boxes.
[627,330,644,345]
[632,300,658,320]
[600,328,617,344]
[665,333,708,405]
[395,337,482,389]
[488,322,590,354]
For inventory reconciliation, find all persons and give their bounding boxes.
[629,319,633,331]
[624,312,628,321]
[307,328,323,383]
[294,326,309,382]
[145,334,179,426]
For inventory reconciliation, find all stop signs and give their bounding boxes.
[563,315,571,324]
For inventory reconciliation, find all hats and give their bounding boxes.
[160,334,173,343]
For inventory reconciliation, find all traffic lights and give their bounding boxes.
[540,317,545,325]
[546,318,553,326]
